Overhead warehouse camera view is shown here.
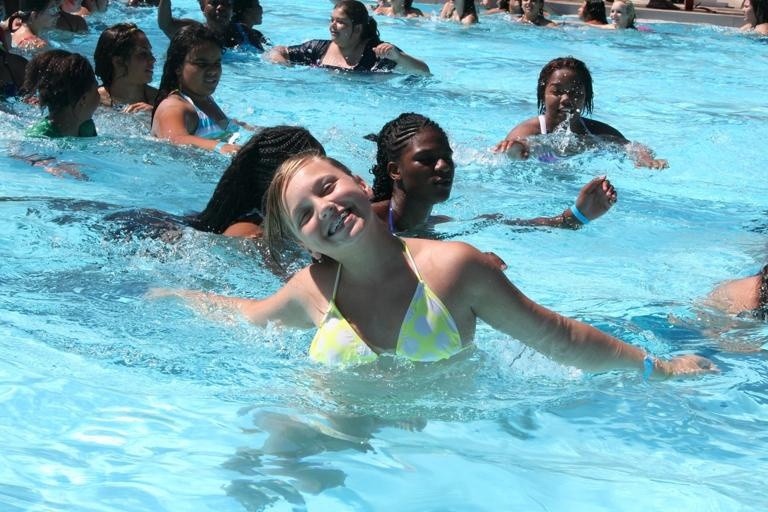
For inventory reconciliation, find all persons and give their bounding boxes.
[1,0,768,376]
